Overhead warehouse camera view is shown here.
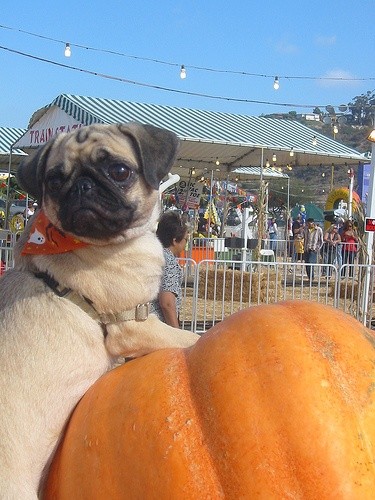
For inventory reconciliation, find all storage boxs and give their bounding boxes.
[228,253,251,272]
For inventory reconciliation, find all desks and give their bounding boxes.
[179,246,214,266]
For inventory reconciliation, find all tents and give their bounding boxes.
[0,127,31,173]
[5,93,370,276]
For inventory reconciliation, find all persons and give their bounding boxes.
[209,221,218,235]
[198,213,208,238]
[268,214,359,282]
[156,210,187,329]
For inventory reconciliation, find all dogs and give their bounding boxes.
[0,122,200,500]
